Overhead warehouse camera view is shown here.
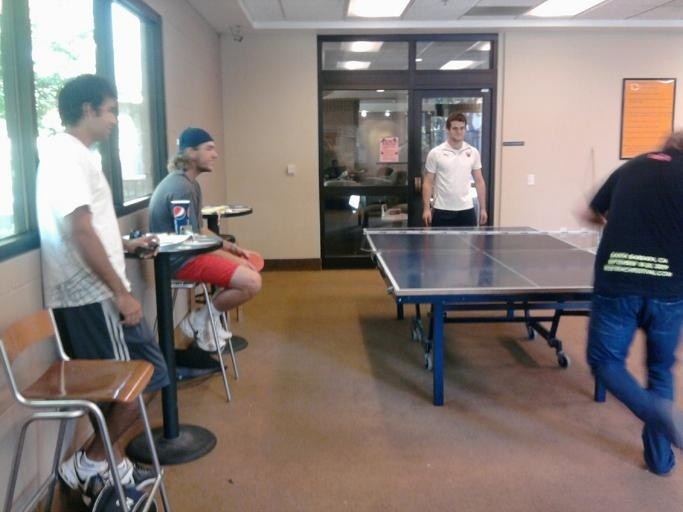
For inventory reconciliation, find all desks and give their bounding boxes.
[121,203,252,466]
[324,177,408,227]
[363,227,599,405]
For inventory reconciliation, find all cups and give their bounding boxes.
[172,199,189,235]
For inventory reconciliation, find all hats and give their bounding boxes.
[176,128,212,155]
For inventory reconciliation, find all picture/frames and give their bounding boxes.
[619,78,677,160]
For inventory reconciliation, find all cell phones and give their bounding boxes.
[136,241,158,259]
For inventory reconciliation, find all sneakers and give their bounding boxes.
[57,447,164,506]
[179,312,233,352]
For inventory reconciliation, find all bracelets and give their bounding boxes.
[229,242,237,253]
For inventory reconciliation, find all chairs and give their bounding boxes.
[0,307,169,511]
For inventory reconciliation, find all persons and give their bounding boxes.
[581,126,683,476]
[421,111,489,227]
[325,159,341,179]
[148,125,263,353]
[36,72,170,511]
